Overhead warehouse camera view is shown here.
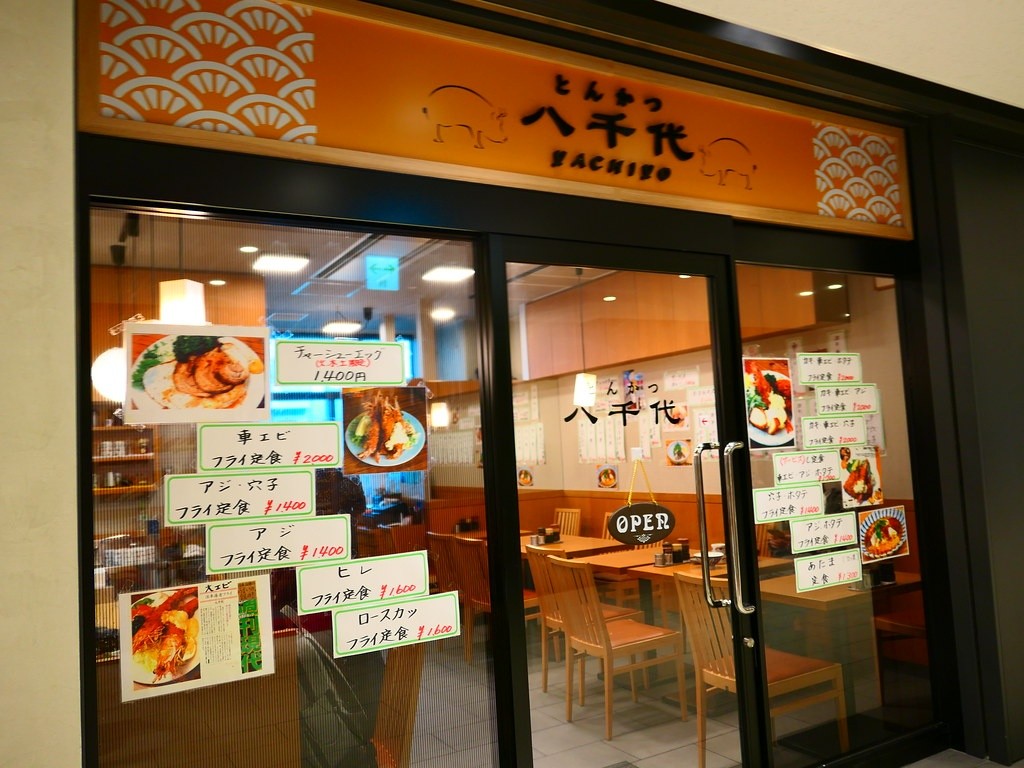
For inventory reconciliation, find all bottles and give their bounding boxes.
[530,524,560,547]
[655,553,664,566]
[678,538,690,561]
[664,554,672,565]
[672,544,683,563]
[663,542,671,553]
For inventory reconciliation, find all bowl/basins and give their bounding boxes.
[667,441,690,465]
[516,468,534,485]
[596,465,617,487]
[694,551,724,569]
[860,508,907,558]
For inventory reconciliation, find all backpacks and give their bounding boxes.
[280,604,387,768]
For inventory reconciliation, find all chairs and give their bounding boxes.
[371,643,426,768]
[426,515,925,768]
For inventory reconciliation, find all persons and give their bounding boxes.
[766,485,896,665]
[269,568,385,768]
[315,468,367,560]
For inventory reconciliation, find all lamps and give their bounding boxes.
[251,251,310,273]
[429,397,451,429]
[91,212,213,402]
[321,311,362,336]
[573,268,596,406]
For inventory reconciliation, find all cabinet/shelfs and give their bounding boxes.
[92,401,161,496]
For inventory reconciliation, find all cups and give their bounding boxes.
[711,543,726,564]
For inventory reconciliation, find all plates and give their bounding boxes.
[130,335,265,409]
[841,456,880,506]
[747,370,794,447]
[130,591,199,683]
[345,411,426,466]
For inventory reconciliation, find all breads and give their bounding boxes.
[750,407,780,435]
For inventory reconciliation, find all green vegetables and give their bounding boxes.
[746,373,778,411]
[351,416,418,447]
[131,335,223,388]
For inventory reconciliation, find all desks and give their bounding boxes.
[759,567,922,762]
[627,555,795,719]
[94,602,302,768]
[486,535,635,662]
[449,530,532,538]
[573,547,711,692]
